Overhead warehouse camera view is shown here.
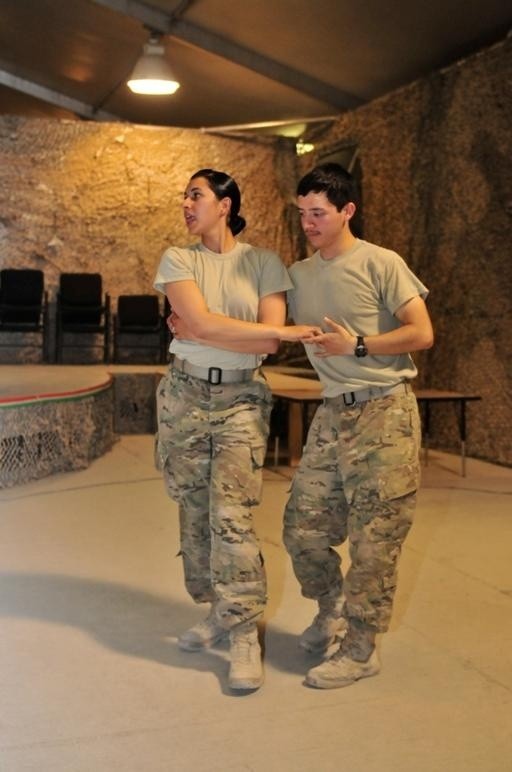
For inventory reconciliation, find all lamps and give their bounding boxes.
[124,23,179,95]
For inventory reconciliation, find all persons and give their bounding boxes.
[154,169,322,697]
[164,167,437,687]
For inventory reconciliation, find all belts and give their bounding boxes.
[172,356,261,385]
[327,382,410,407]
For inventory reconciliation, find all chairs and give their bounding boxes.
[1,268,51,364]
[56,270,113,366]
[110,293,164,362]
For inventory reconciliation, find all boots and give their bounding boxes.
[305,619,381,689]
[176,613,228,652]
[228,625,263,690]
[297,613,348,654]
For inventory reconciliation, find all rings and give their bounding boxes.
[170,327,176,333]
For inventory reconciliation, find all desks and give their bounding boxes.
[267,388,481,477]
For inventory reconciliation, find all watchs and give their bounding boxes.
[355,336,368,359]
